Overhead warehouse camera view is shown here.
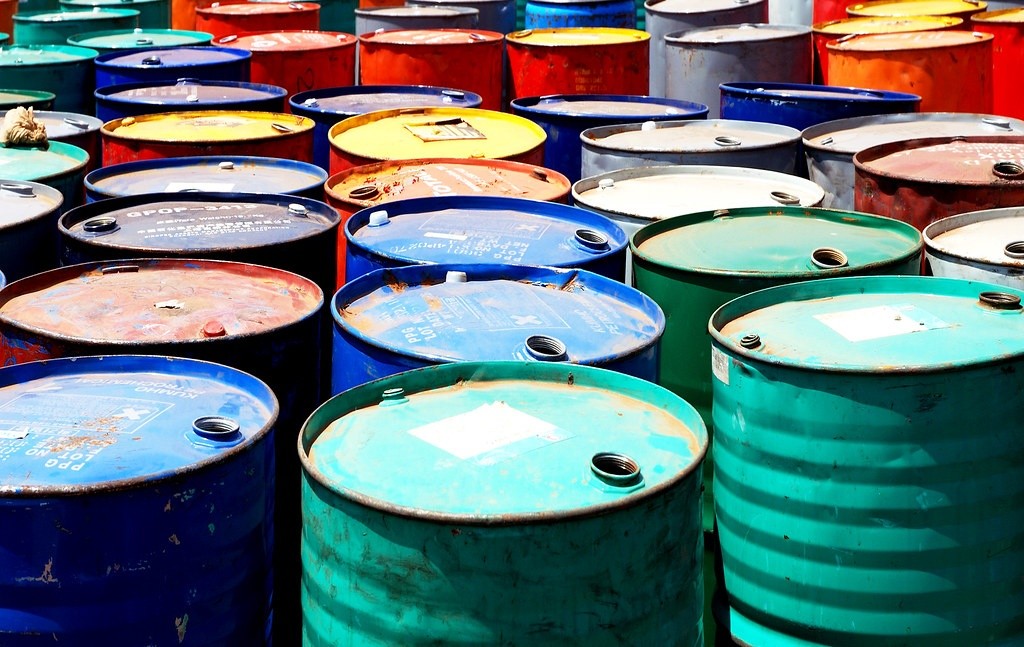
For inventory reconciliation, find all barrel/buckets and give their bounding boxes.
[0,0,1024,647]
